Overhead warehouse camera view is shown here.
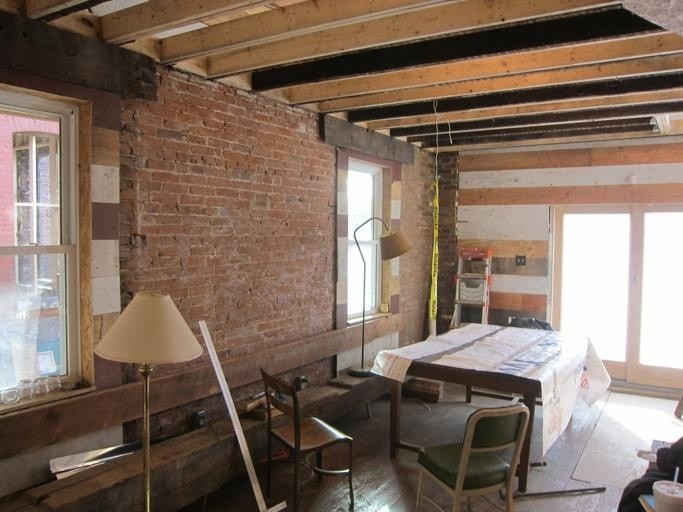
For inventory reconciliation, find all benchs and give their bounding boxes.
[1,313,413,512]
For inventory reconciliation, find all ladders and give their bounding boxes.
[453,247,493,329]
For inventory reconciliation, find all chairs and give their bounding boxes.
[259,366,356,511]
[414,403,532,512]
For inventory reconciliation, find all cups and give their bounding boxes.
[650,479,683,512]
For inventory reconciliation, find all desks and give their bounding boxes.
[389,321,591,492]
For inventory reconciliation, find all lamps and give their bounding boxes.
[348,216,414,377]
[93,291,205,512]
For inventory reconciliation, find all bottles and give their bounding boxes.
[0,373,71,405]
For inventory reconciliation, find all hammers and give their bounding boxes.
[499,485,606,499]
